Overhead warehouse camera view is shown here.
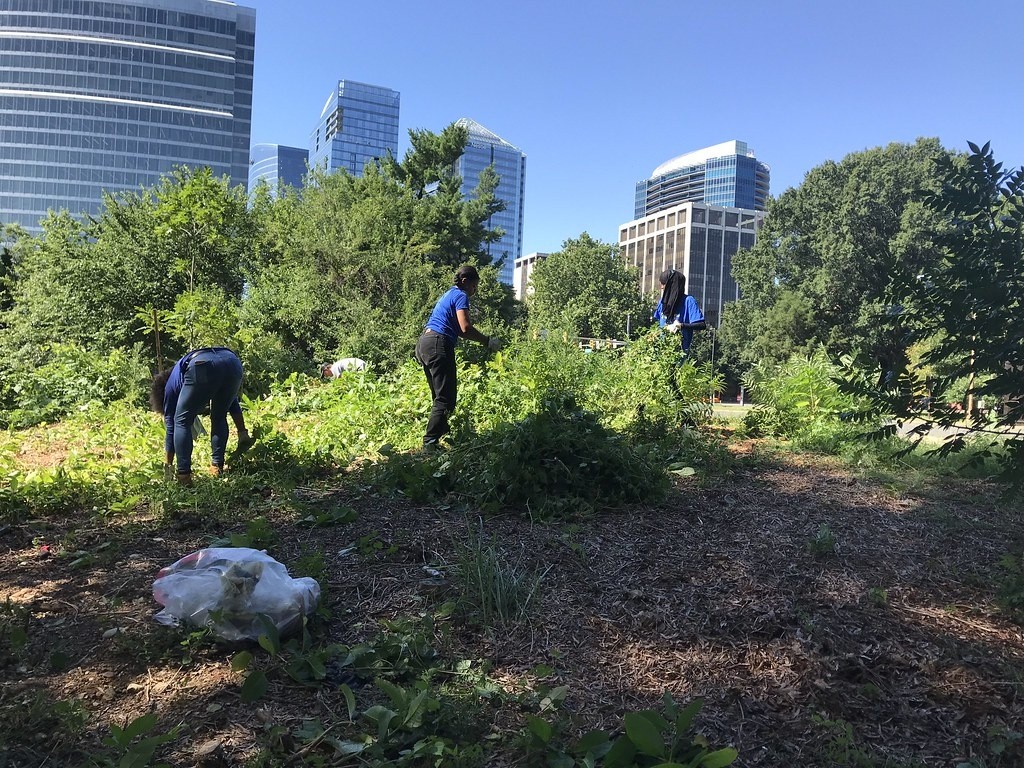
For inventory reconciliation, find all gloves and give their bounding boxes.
[488,337,502,352]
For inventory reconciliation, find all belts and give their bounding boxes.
[424,328,445,338]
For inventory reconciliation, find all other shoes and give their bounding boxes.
[208,464,223,478]
[172,474,193,488]
[426,439,447,457]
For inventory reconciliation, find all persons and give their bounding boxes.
[319,357,367,379]
[150,346,251,487]
[415,265,504,451]
[654,269,707,429]
